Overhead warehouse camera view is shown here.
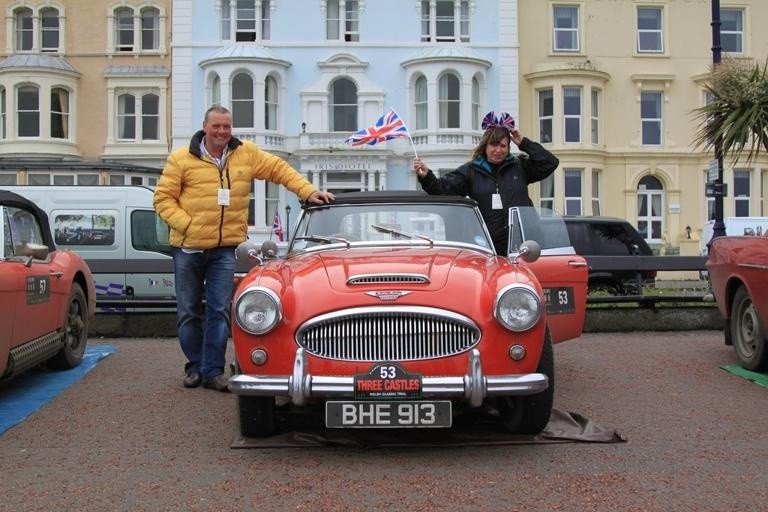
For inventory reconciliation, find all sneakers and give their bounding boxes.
[203,374,230,392]
[183,372,201,387]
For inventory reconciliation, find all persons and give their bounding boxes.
[151,105,336,393]
[413,110,559,259]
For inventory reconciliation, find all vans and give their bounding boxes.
[1,184,206,316]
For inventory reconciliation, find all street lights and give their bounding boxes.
[285,205,291,241]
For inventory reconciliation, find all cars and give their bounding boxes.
[0,189,96,384]
[229,190,589,437]
[538,214,656,295]
[707,228,768,371]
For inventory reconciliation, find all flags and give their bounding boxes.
[344,109,408,147]
[273,213,282,242]
